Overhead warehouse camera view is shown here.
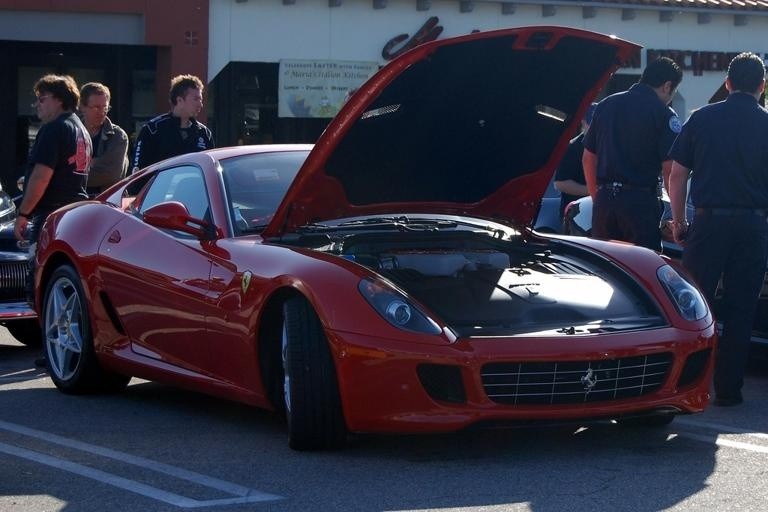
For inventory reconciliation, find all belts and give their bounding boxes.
[695,206,768,218]
[603,180,656,192]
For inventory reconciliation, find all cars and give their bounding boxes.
[169,273,222,300]
[1,181,44,359]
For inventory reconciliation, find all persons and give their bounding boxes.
[579,55,685,257]
[76,82,129,195]
[669,50,768,407]
[130,73,217,174]
[553,102,599,234]
[14,72,95,367]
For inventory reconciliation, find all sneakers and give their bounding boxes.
[34,357,48,368]
[714,394,744,407]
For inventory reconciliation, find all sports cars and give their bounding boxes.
[38,23,715,458]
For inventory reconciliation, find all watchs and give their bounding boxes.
[15,209,31,220]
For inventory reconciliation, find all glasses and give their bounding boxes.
[37,94,54,105]
[84,100,112,114]
